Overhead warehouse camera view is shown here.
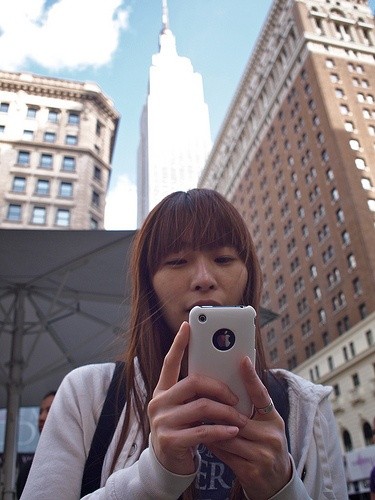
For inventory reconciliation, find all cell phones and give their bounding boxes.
[186,305,257,421]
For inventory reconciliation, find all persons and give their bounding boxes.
[16,390,57,500]
[23,190,350,500]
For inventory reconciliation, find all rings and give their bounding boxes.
[256,398,274,414]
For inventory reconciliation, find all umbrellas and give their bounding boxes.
[0,229,280,500]
